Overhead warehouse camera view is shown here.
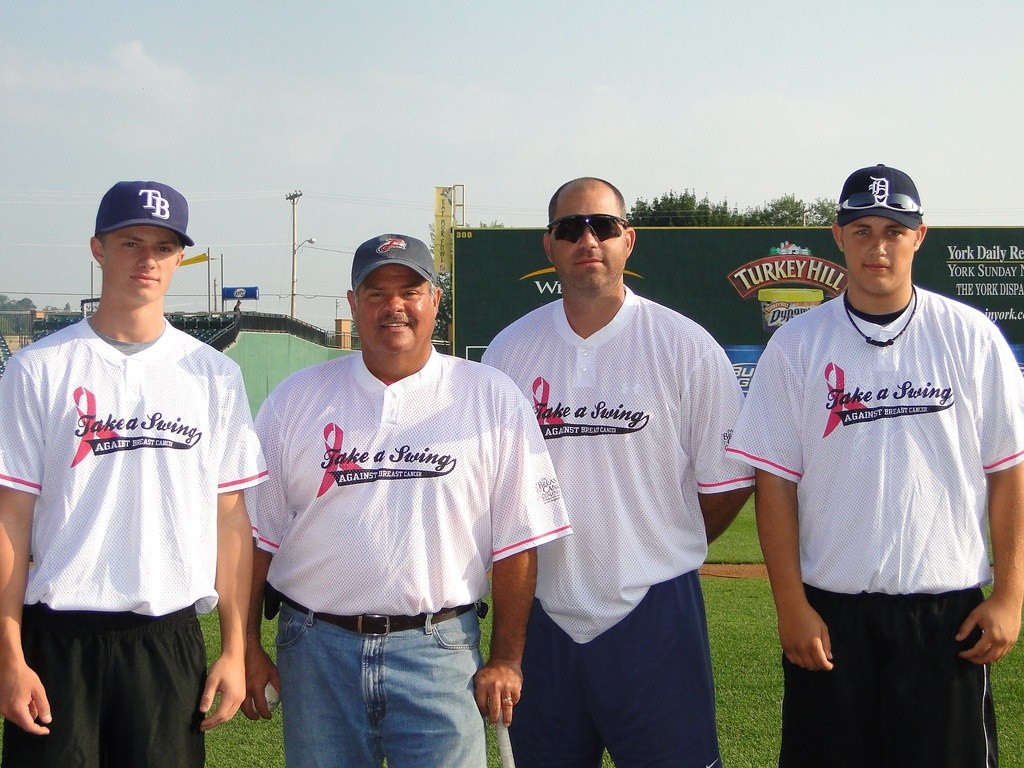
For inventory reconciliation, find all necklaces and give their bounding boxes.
[843,286,919,348]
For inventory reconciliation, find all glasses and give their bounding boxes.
[840,192,922,215]
[548,214,630,243]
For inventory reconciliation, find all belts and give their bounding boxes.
[280,588,489,637]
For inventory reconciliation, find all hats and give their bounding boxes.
[838,163,923,232]
[351,232,436,291]
[95,181,195,247]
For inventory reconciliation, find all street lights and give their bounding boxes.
[290,238,317,322]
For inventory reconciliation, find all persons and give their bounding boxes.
[240,234,572,768]
[0,179,271,768]
[482,174,758,768]
[724,161,1024,768]
